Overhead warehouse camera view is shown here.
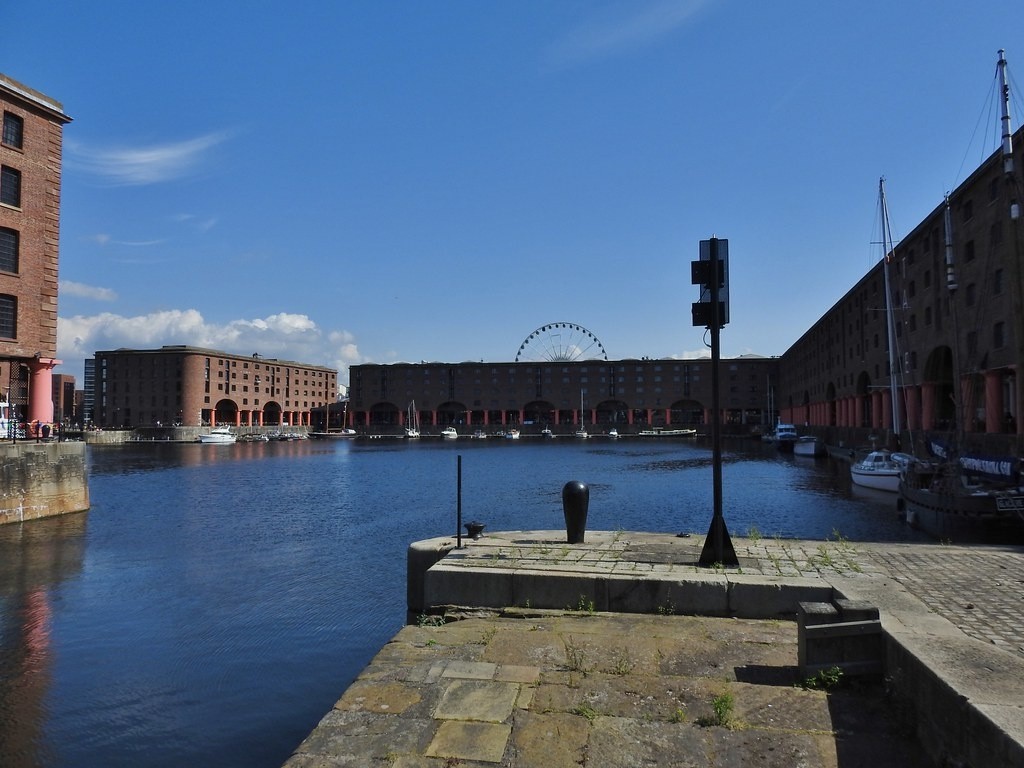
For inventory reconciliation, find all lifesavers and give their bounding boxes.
[30,419,39,434]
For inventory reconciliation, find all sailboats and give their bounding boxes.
[575,389,587,438]
[403,400,421,438]
[851,171,1024,544]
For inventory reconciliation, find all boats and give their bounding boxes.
[506,414,520,440]
[198,424,309,443]
[609,428,622,438]
[751,374,799,445]
[471,430,487,439]
[793,436,825,457]
[440,426,458,439]
[639,427,696,437]
[541,425,552,440]
